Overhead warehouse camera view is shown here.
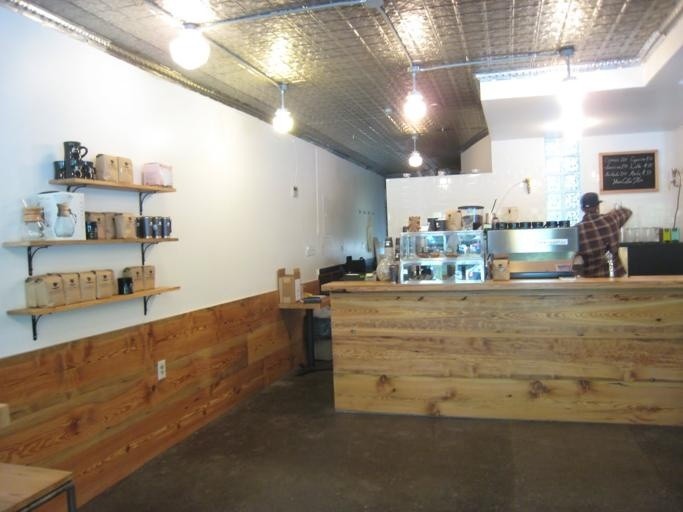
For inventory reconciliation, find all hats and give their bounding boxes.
[580,192,603,210]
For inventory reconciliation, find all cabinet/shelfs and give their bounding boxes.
[3,177,181,341]
[399,229,486,283]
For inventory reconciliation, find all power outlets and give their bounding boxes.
[157,359,166,381]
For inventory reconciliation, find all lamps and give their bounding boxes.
[557,47,584,102]
[402,64,426,121]
[272,83,293,133]
[408,135,423,167]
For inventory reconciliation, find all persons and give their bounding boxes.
[573,192,633,278]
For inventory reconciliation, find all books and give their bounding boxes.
[304,297,320,304]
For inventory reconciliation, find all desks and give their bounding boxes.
[277,296,332,376]
[0,462,76,512]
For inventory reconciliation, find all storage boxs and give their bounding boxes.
[276,268,301,304]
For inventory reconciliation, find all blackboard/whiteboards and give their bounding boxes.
[598,149,659,194]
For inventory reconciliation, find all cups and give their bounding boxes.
[557,220,570,228]
[520,222,531,229]
[544,221,557,228]
[53,141,96,179]
[496,222,506,229]
[118,278,133,294]
[532,222,543,228]
[509,223,518,228]
[86,221,98,240]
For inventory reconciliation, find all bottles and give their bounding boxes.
[390,265,399,284]
[137,217,172,239]
